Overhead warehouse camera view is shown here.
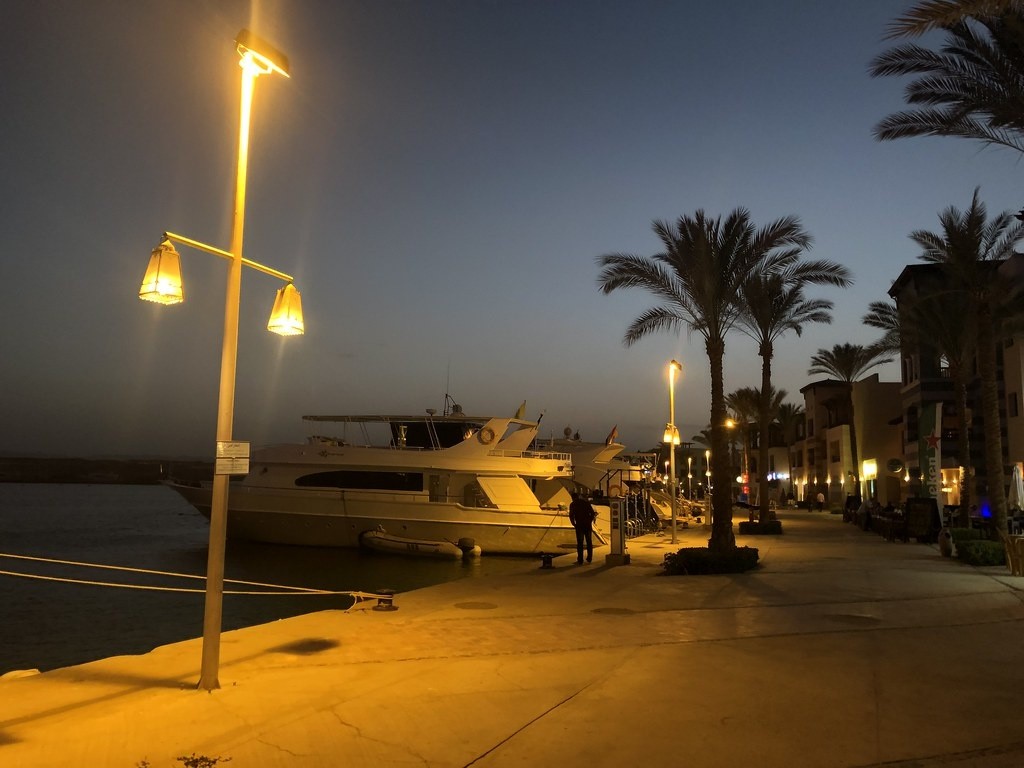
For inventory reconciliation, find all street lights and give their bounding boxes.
[141,29,307,692]
[663,360,754,545]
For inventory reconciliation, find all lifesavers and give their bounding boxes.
[680,500,693,514]
[478,426,495,444]
[608,484,622,498]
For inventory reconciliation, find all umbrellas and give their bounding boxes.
[1008,465,1024,511]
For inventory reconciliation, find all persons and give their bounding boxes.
[807,491,814,513]
[857,497,904,532]
[770,497,777,509]
[817,491,825,513]
[568,493,595,565]
[786,490,794,511]
[1012,499,1021,511]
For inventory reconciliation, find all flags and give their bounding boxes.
[918,402,943,498]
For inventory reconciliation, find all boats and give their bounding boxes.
[526,442,705,541]
[359,529,483,560]
[159,364,610,554]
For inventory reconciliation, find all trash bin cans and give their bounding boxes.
[749,505,761,523]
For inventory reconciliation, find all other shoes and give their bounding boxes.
[578,558,582,564]
[586,557,592,563]
